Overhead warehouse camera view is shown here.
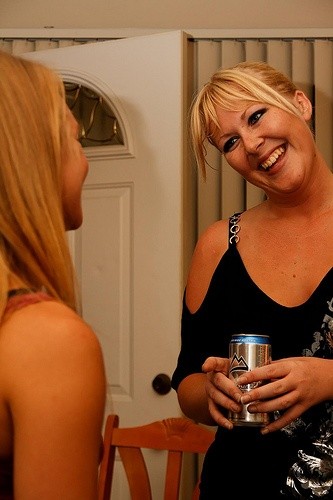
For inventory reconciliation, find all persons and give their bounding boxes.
[0,51,106,500]
[171,61,333,500]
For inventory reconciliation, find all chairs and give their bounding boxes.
[98,416,216,500]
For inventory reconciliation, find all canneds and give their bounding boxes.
[226,334,275,427]
